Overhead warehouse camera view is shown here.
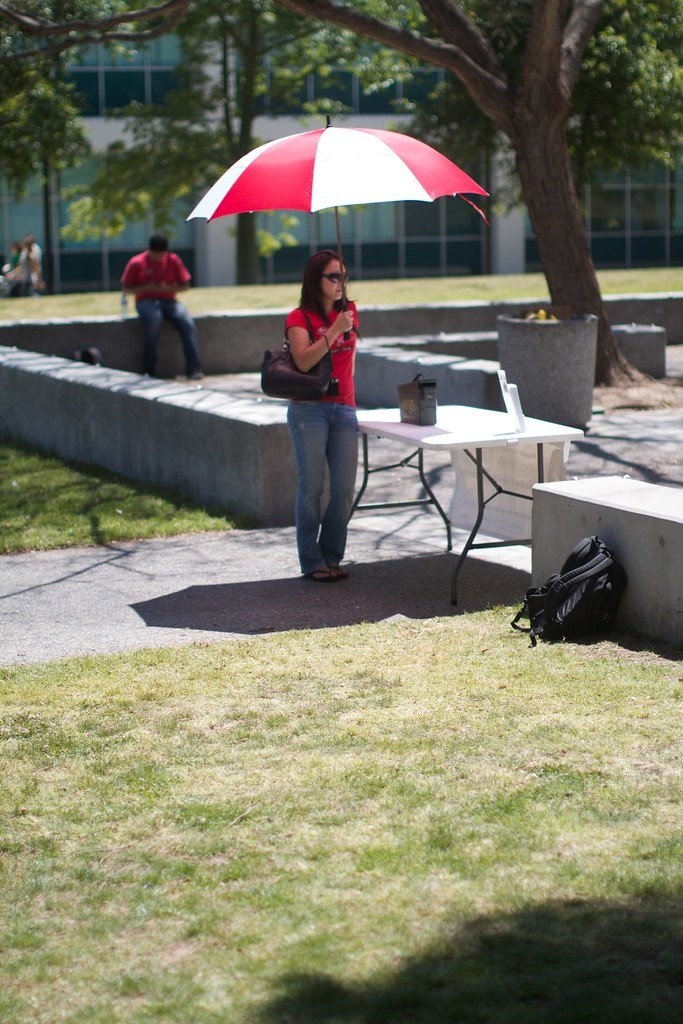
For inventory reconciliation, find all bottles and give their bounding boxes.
[120,293,127,318]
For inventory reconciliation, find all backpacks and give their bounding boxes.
[510,536,627,648]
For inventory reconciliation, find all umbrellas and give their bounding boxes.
[184,117,489,340]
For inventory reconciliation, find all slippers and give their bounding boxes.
[304,565,349,583]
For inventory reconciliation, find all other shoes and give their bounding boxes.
[185,368,203,379]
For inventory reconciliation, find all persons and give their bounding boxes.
[284,250,360,581]
[120,234,207,378]
[0,235,43,295]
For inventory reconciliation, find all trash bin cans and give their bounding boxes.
[493,309,597,430]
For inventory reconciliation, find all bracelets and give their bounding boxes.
[324,335,330,351]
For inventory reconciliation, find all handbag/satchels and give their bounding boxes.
[261,308,332,401]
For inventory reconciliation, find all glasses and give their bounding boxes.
[321,272,350,283]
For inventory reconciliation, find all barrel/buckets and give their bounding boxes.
[497,312,597,427]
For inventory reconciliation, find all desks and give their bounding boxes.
[347,404,584,606]
